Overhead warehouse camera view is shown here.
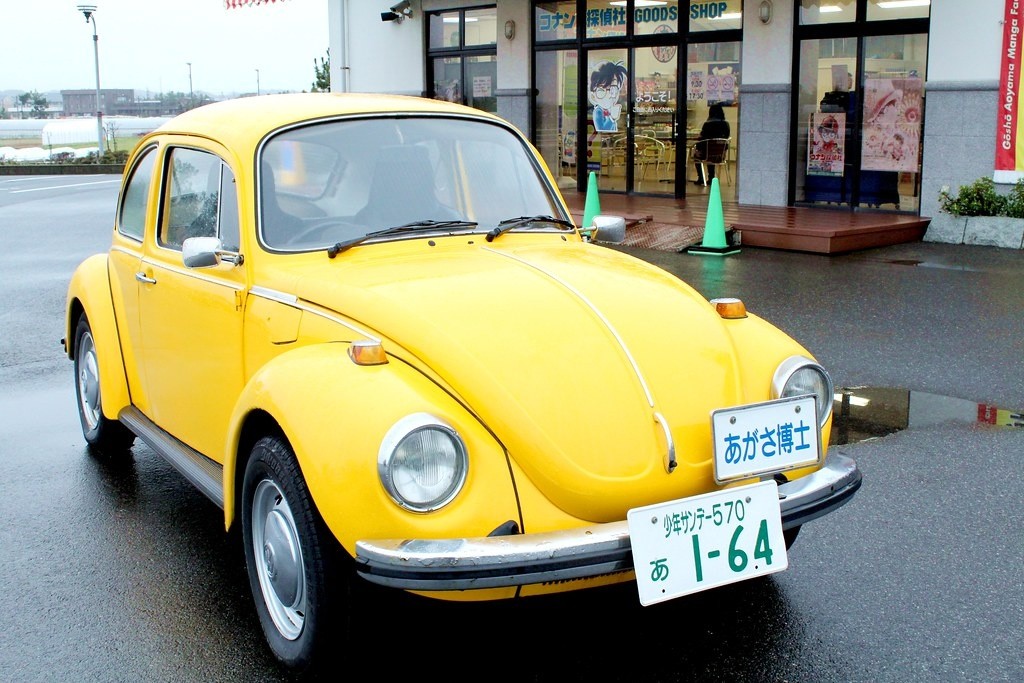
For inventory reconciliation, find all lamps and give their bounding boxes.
[504,20,516,40]
[758,0,772,24]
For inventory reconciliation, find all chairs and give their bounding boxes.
[601,130,732,187]
[184,160,310,253]
[355,145,461,228]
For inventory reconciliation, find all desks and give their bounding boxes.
[653,137,700,170]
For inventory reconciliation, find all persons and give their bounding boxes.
[693,104,730,186]
[846,73,854,91]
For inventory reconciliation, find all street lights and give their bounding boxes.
[186,63,194,107]
[256,69,259,95]
[77,5,104,158]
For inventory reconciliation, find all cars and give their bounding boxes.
[60,93,865,673]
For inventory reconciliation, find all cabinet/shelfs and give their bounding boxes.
[617,108,695,130]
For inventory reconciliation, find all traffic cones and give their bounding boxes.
[688,177,741,257]
[581,172,601,235]
